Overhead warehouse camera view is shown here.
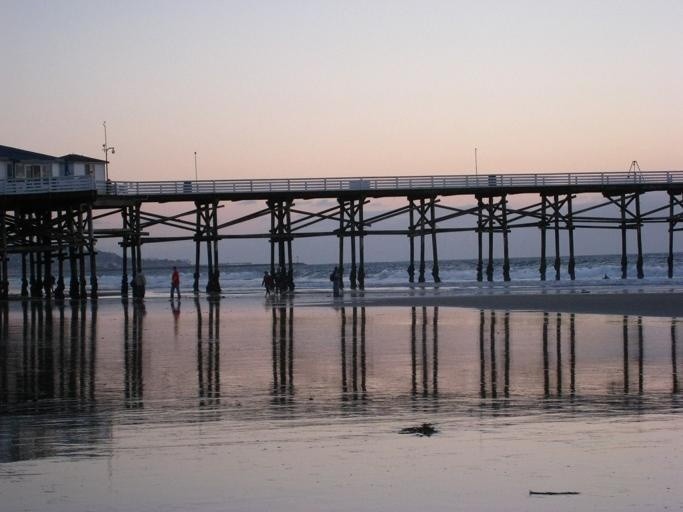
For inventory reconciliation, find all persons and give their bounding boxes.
[0,269,147,302]
[168,265,182,302]
[261,265,366,298]
